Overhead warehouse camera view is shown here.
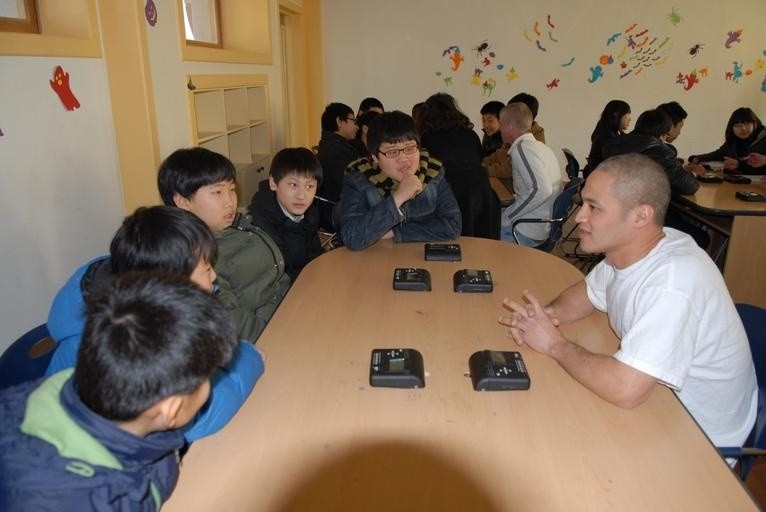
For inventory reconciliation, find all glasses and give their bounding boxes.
[378,145,417,158]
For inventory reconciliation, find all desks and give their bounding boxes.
[159,235,766,511]
[673,158,766,308]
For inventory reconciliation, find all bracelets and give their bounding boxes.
[690,170,698,177]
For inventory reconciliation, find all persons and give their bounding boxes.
[251,148,325,282]
[629,101,684,166]
[315,96,385,250]
[156,146,291,344]
[722,150,766,176]
[0,265,238,511]
[688,107,766,171]
[410,93,498,238]
[341,112,461,250]
[480,93,558,247]
[579,100,632,196]
[497,152,759,470]
[601,107,712,250]
[46,206,264,442]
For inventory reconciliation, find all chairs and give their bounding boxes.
[509,145,604,278]
[0,321,58,388]
[714,301,766,478]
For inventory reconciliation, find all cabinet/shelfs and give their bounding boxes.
[187,74,278,205]
